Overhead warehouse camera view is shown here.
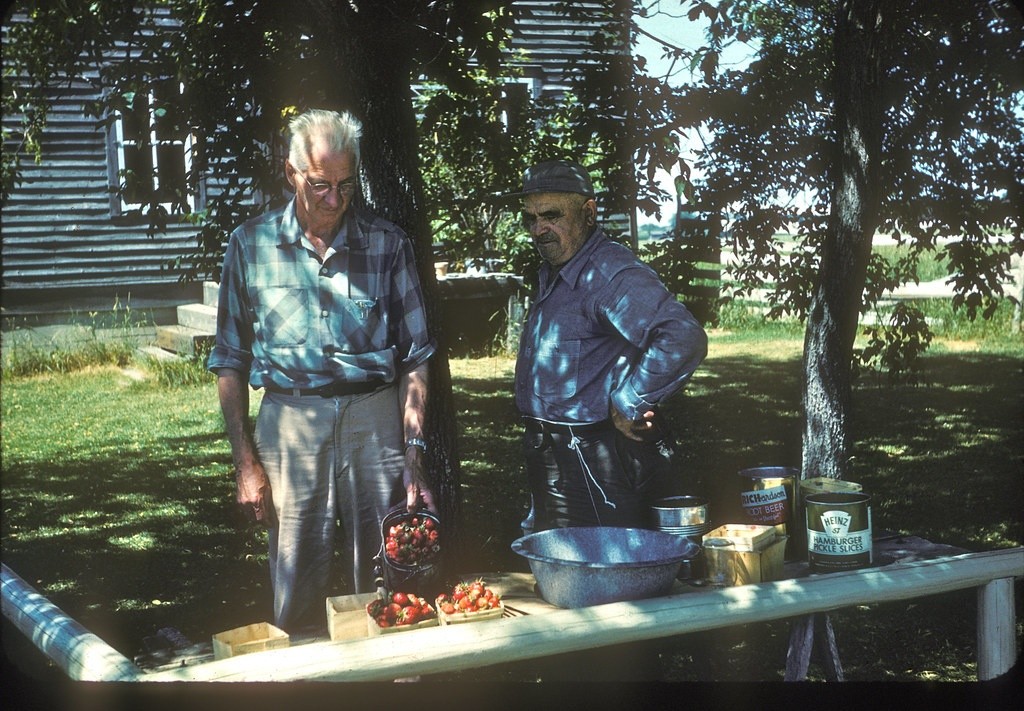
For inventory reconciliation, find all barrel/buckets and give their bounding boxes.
[371,507,448,596]
[649,496,716,578]
[805,492,874,574]
[737,467,801,546]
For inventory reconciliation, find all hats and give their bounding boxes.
[501,159,596,198]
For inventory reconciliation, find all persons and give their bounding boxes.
[208,109,438,628]
[501,161,707,682]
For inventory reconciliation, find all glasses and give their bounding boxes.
[295,167,356,196]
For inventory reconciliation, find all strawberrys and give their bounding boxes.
[368,591,436,627]
[438,577,502,613]
[385,517,438,564]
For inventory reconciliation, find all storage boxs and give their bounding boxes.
[326,592,394,642]
[701,524,788,587]
[373,508,448,608]
[366,591,439,638]
[435,577,505,627]
[212,621,290,661]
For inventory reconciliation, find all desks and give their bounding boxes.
[133,529,979,682]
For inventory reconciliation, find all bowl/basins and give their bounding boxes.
[510,526,705,609]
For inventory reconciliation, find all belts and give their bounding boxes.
[268,379,386,399]
[522,416,616,452]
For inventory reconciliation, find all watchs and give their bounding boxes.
[403,438,428,455]
